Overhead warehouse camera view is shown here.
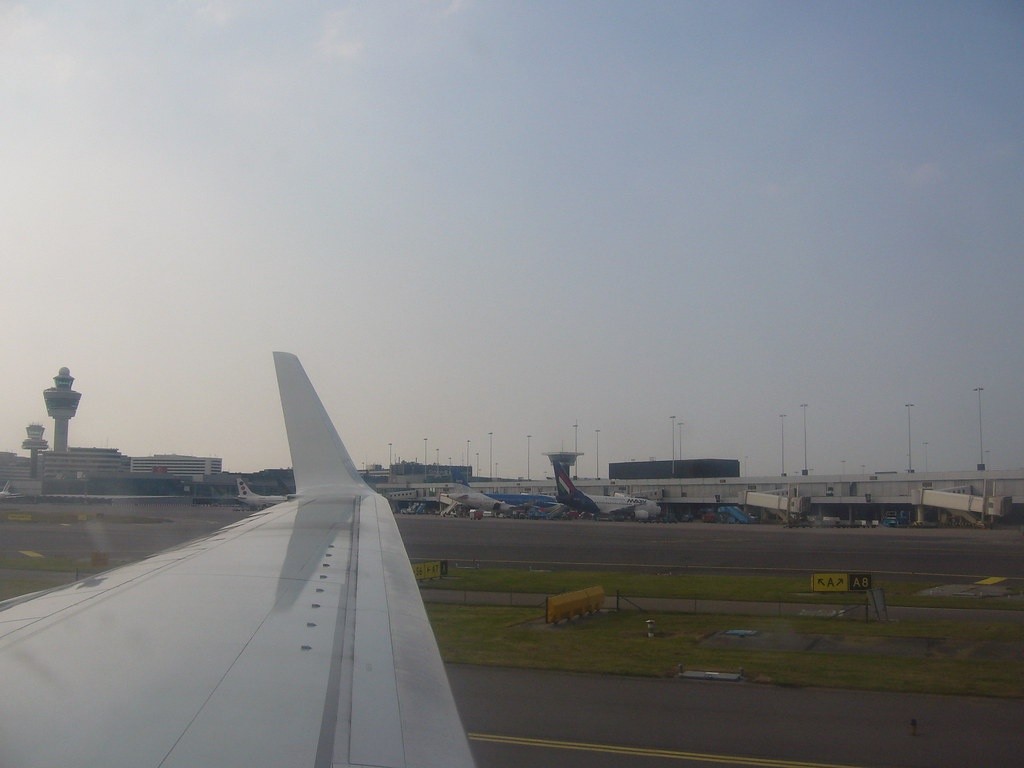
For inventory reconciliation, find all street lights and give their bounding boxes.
[423,438,427,483]
[670,415,676,478]
[527,434,532,479]
[466,439,471,483]
[595,428,602,480]
[489,433,493,481]
[973,387,986,464]
[572,425,579,479]
[780,414,787,474]
[903,403,914,475]
[677,422,683,460]
[476,453,480,480]
[436,448,440,475]
[388,443,392,465]
[800,403,810,470]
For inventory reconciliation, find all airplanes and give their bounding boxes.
[440,483,516,519]
[235,479,286,510]
[1,351,474,768]
[484,460,663,523]
